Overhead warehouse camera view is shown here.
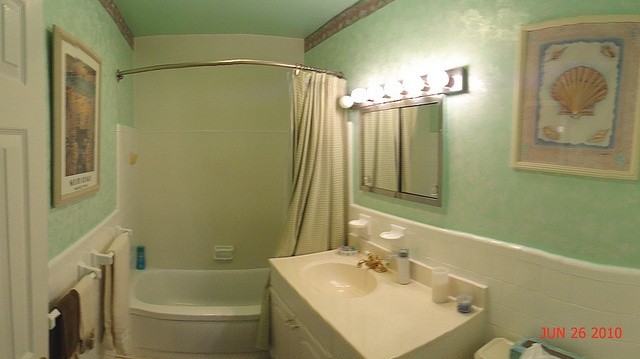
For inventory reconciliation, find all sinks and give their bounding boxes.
[294,260,379,297]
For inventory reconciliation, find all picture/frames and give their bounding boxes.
[511,15,637,180]
[50,24,103,207]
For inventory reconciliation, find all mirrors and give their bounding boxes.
[358,94,445,208]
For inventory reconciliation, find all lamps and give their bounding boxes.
[339,66,464,109]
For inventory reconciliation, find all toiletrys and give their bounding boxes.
[133,244,147,270]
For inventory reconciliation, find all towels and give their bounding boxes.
[53,288,80,357]
[103,233,134,357]
[71,274,100,343]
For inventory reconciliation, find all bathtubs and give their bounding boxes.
[130,268,267,354]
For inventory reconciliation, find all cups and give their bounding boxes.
[432,266,448,304]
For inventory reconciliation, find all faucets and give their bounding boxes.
[356,258,388,274]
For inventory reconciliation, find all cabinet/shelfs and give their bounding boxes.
[267,287,333,359]
